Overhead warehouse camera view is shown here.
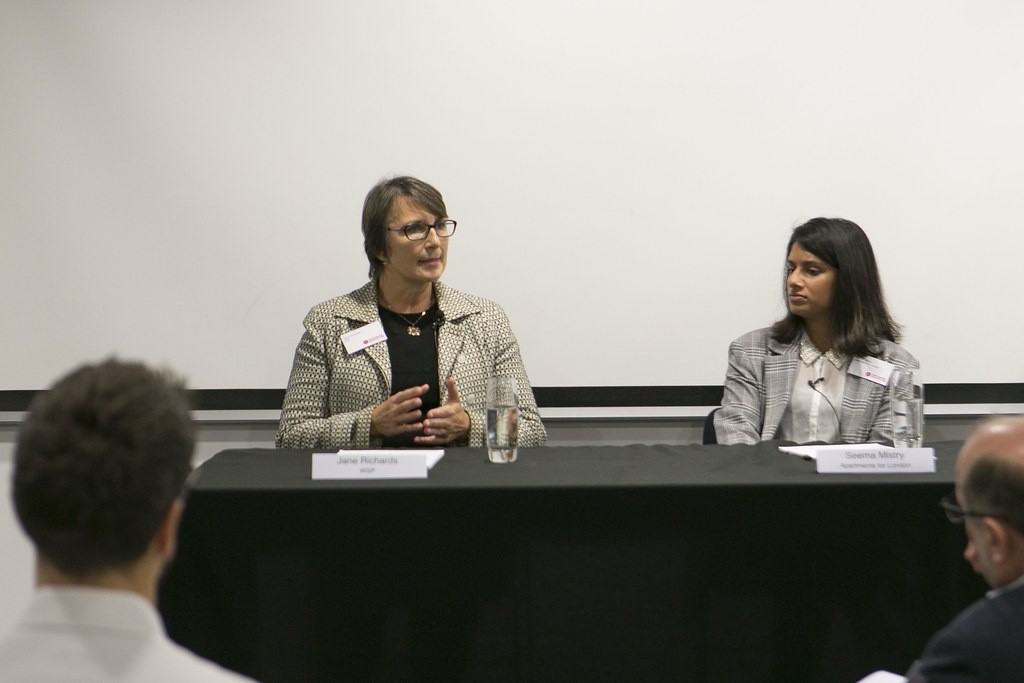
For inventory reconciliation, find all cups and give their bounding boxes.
[893,369,922,451]
[484,378,521,462]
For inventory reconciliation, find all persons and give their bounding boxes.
[905,416,1024,683]
[712,217,924,447]
[0,356,258,683]
[274,174,548,447]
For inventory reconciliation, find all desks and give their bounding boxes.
[156,440,990,683]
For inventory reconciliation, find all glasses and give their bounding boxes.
[940,491,1015,525]
[382,220,456,241]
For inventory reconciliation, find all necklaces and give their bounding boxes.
[379,293,435,336]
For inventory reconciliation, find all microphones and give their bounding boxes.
[809,380,816,390]
[437,310,444,319]
